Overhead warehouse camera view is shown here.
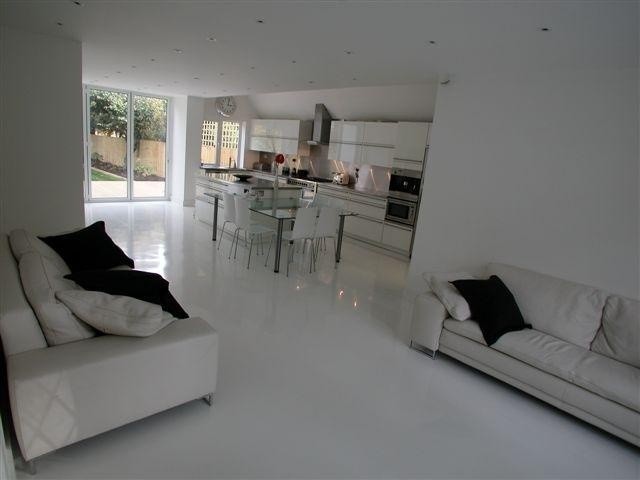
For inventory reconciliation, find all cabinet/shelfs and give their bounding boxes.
[247,118,299,155]
[392,121,427,171]
[317,188,386,246]
[381,222,413,252]
[328,121,398,170]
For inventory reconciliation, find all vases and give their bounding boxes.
[273,166,279,200]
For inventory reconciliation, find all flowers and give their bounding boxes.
[274,153,285,166]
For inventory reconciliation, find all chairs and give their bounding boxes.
[216,190,339,278]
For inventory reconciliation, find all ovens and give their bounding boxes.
[384,166,422,229]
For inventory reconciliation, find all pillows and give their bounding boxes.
[422,270,533,348]
[38,220,190,338]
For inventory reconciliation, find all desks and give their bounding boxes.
[204,191,359,272]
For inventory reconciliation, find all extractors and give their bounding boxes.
[298,103,332,146]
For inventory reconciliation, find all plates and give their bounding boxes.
[234,175,253,181]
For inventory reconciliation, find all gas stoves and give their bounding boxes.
[290,176,333,182]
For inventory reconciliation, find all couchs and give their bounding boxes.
[408,260,639,447]
[0,225,220,475]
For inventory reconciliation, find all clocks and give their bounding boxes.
[215,96,237,117]
